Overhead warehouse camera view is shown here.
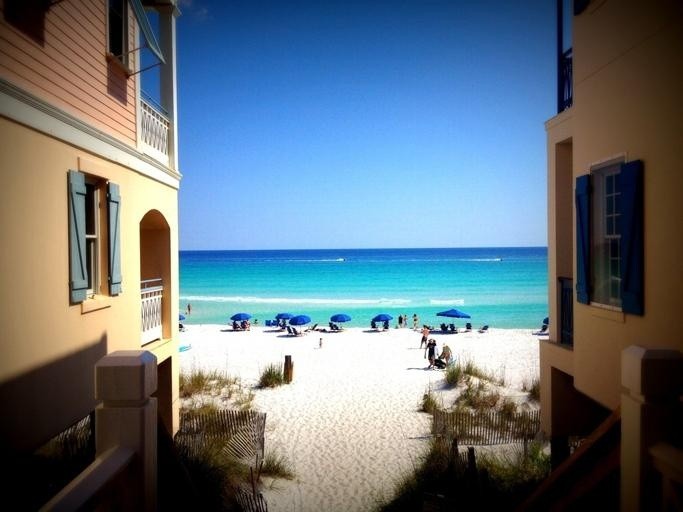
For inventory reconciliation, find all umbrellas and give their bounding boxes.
[372,313,392,321]
[287,314,312,332]
[178,314,186,321]
[330,314,351,328]
[229,313,251,321]
[435,309,472,319]
[274,313,294,319]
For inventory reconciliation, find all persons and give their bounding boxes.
[403,314,408,326]
[420,324,429,349]
[440,342,452,368]
[233,320,250,329]
[424,339,436,370]
[398,313,403,327]
[411,314,419,329]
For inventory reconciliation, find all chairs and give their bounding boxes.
[440,323,457,334]
[466,323,472,331]
[371,321,389,331]
[264,319,344,337]
[481,325,488,332]
[232,322,250,330]
[532,324,549,336]
[179,323,184,329]
